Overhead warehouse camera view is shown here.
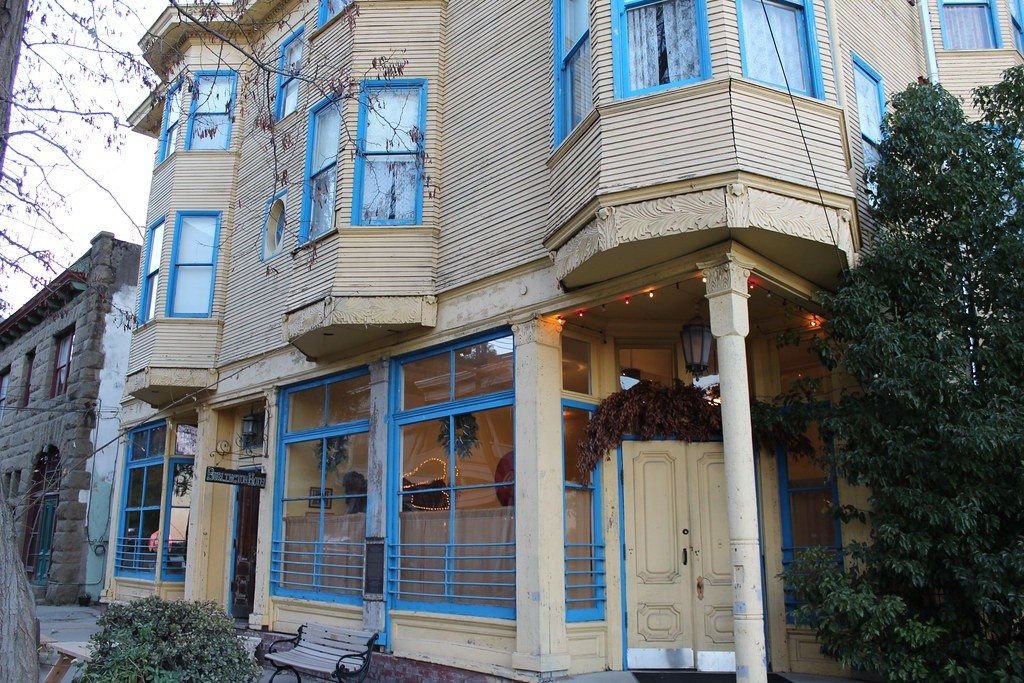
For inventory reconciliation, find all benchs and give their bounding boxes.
[265,622,379,683]
[44,641,104,683]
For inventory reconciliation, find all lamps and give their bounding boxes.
[681,315,713,381]
[242,416,260,443]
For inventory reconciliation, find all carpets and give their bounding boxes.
[632,671,793,683]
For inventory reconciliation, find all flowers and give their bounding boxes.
[437,413,481,461]
[316,434,349,473]
[576,379,816,487]
